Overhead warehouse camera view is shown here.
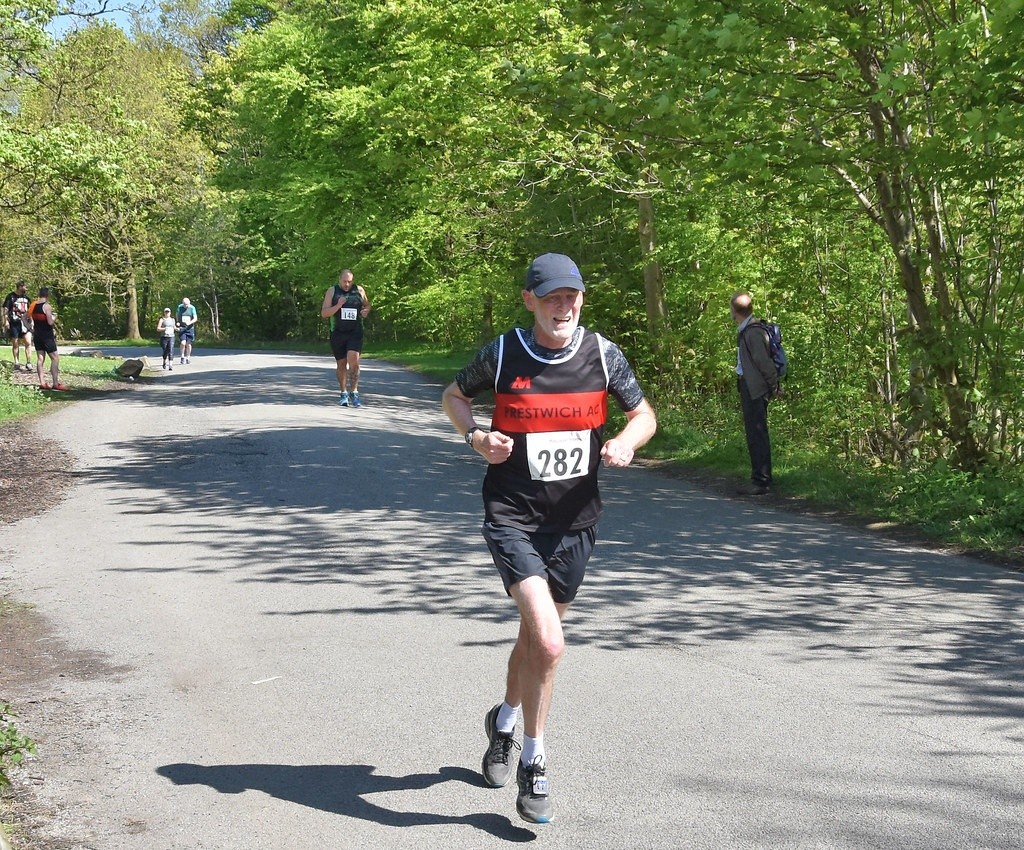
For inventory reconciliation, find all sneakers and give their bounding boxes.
[482,704,522,787]
[516,752,556,823]
[52,383,68,390]
[26,363,33,370]
[14,363,20,369]
[340,392,348,406]
[349,392,361,406]
[40,382,52,389]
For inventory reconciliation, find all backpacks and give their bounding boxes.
[738,320,787,377]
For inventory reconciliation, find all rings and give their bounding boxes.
[620,456,626,461]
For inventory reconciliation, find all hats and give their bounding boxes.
[526,253,586,298]
[164,308,171,312]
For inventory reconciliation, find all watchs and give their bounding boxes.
[465,426,482,448]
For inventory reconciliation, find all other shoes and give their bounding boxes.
[163,363,166,369]
[181,358,184,363]
[736,484,771,494]
[186,359,190,364]
[169,366,172,370]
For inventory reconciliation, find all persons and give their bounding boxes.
[176,297,198,365]
[320,268,372,408]
[2,280,35,371]
[23,287,67,390]
[442,253,657,823]
[730,292,782,494]
[157,307,180,371]
